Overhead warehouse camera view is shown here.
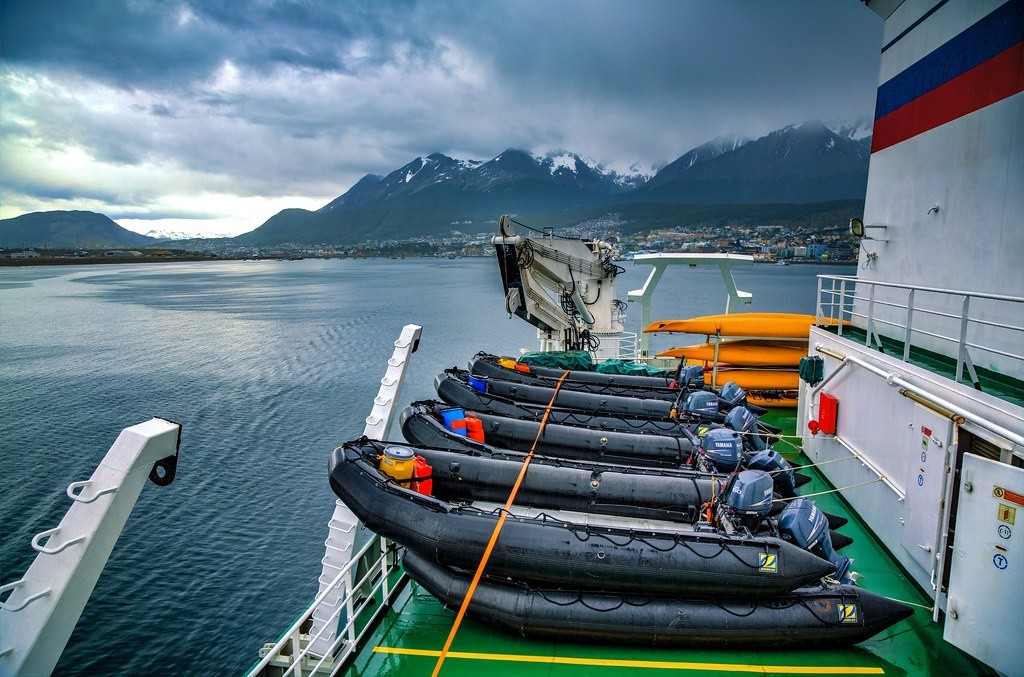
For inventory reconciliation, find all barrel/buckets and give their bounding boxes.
[440,406,467,437]
[469,373,489,393]
[379,445,415,489]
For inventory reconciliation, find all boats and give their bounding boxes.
[778,261,789,265]
[321,436,844,592]
[753,253,778,264]
[704,368,799,391]
[464,352,771,417]
[642,313,852,342]
[396,394,826,488]
[652,338,809,368]
[396,544,918,660]
[745,395,799,406]
[435,368,782,450]
